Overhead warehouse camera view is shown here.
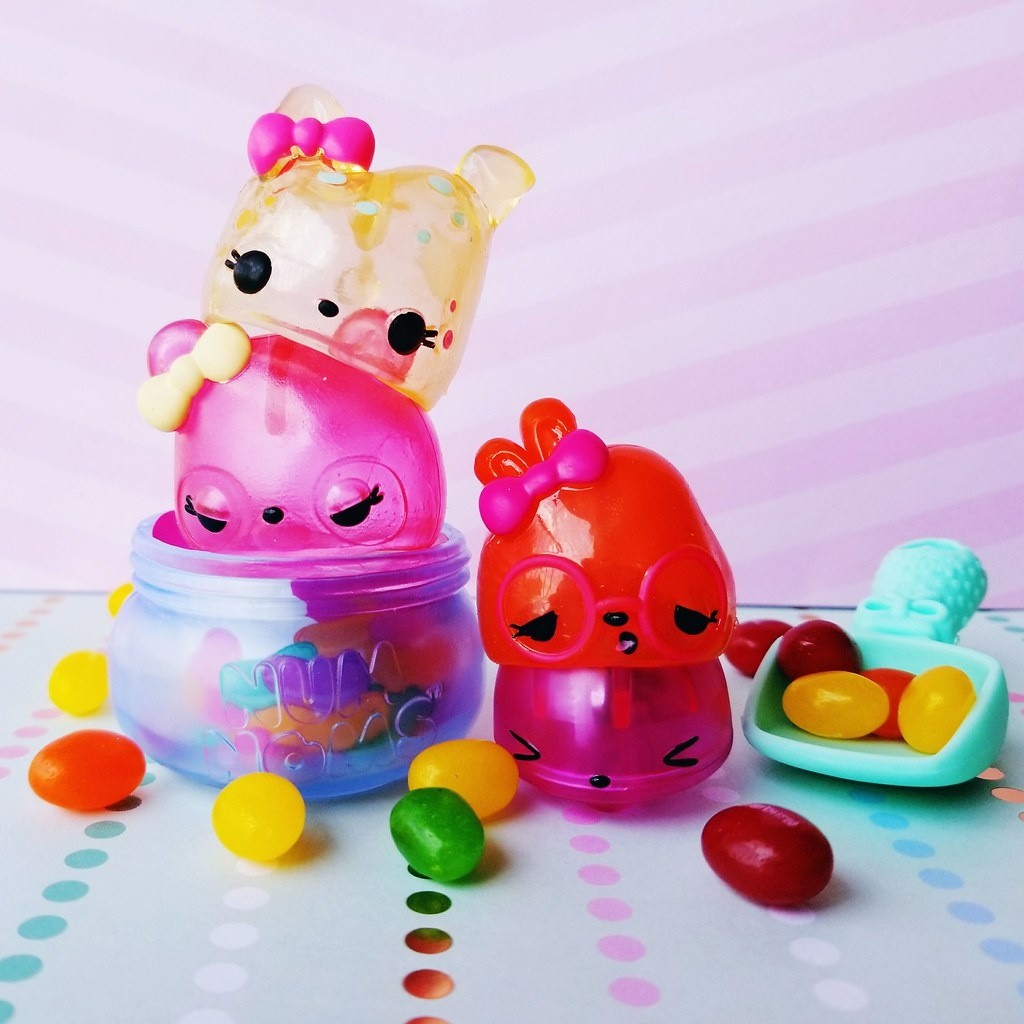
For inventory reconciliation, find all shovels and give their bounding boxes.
[735,532,1010,790]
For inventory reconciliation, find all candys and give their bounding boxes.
[26,615,977,905]
